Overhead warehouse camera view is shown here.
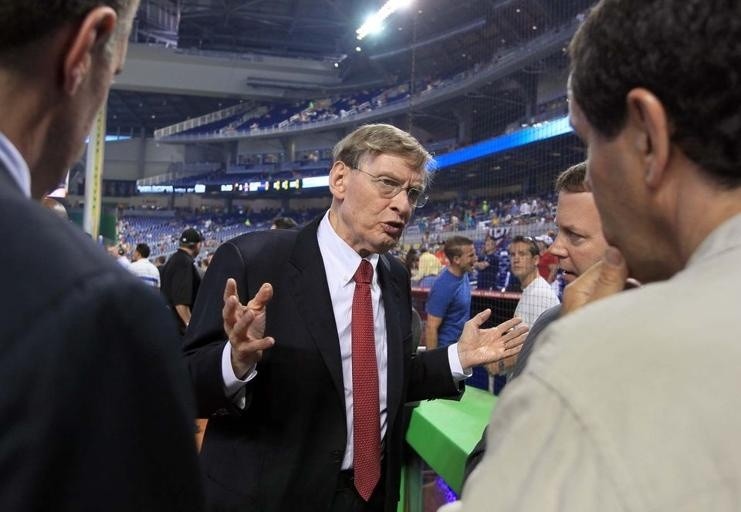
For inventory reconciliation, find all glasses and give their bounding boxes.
[354,167,429,208]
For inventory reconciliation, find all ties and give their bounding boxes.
[352,260,381,502]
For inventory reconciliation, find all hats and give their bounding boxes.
[179,228,202,242]
[535,235,553,246]
